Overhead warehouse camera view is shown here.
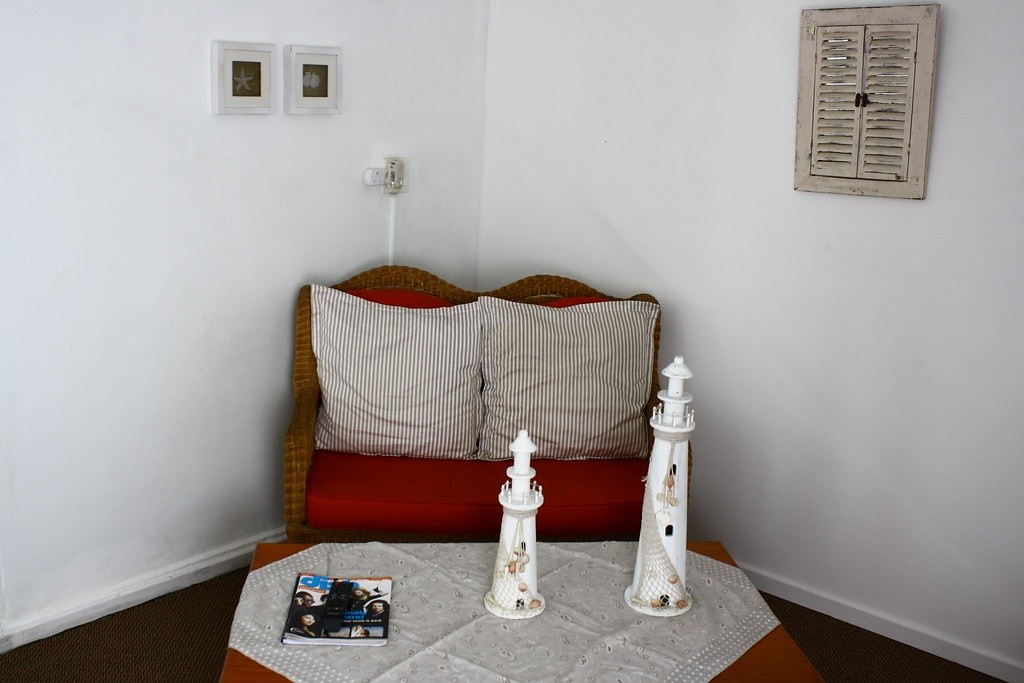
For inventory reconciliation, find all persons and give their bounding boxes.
[287,589,389,638]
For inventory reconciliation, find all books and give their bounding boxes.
[281,573,392,647]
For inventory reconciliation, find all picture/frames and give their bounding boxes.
[283,44,343,116]
[211,40,278,116]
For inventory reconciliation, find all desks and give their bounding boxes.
[216,543,825,683]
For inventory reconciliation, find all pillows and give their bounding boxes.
[476,295,661,462]
[310,283,486,461]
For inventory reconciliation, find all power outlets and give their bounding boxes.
[363,168,387,186]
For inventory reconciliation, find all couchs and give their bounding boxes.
[283,265,693,542]
